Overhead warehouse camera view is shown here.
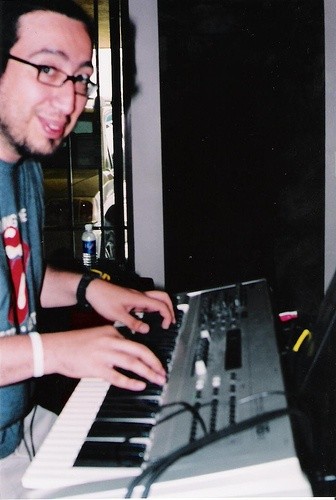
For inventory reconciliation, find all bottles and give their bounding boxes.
[81,223,97,267]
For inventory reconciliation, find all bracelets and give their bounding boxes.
[29,330,43,378]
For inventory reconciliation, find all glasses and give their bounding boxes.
[8,54,98,96]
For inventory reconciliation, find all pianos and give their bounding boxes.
[21,278,314,497]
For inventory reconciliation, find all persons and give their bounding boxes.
[0,0,176,499]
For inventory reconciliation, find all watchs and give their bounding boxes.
[76,268,112,309]
